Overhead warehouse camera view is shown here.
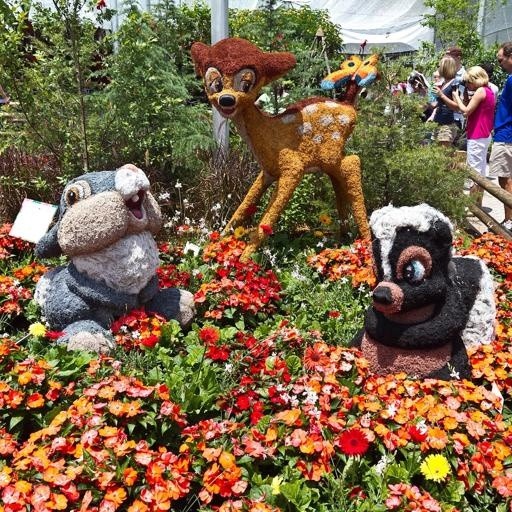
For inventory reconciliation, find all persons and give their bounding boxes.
[384,41,512,232]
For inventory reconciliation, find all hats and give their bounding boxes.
[446,47,462,56]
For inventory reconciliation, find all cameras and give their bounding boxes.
[409,75,427,90]
[451,82,459,92]
